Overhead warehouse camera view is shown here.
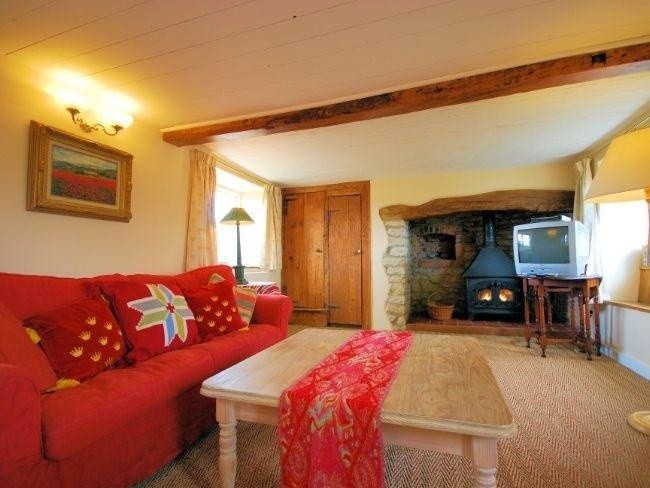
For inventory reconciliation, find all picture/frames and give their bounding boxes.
[26,119,136,223]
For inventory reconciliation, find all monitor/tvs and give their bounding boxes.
[514,220,589,279]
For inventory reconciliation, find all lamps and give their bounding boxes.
[583,126,650,437]
[50,76,134,136]
[220,208,256,286]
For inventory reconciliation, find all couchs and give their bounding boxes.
[1,265,292,488]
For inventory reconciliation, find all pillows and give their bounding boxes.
[93,276,202,360]
[23,295,129,384]
[208,273,255,331]
[180,280,256,340]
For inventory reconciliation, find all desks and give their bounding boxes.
[522,274,602,360]
[200,327,516,488]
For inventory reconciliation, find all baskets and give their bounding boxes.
[428,302,455,320]
